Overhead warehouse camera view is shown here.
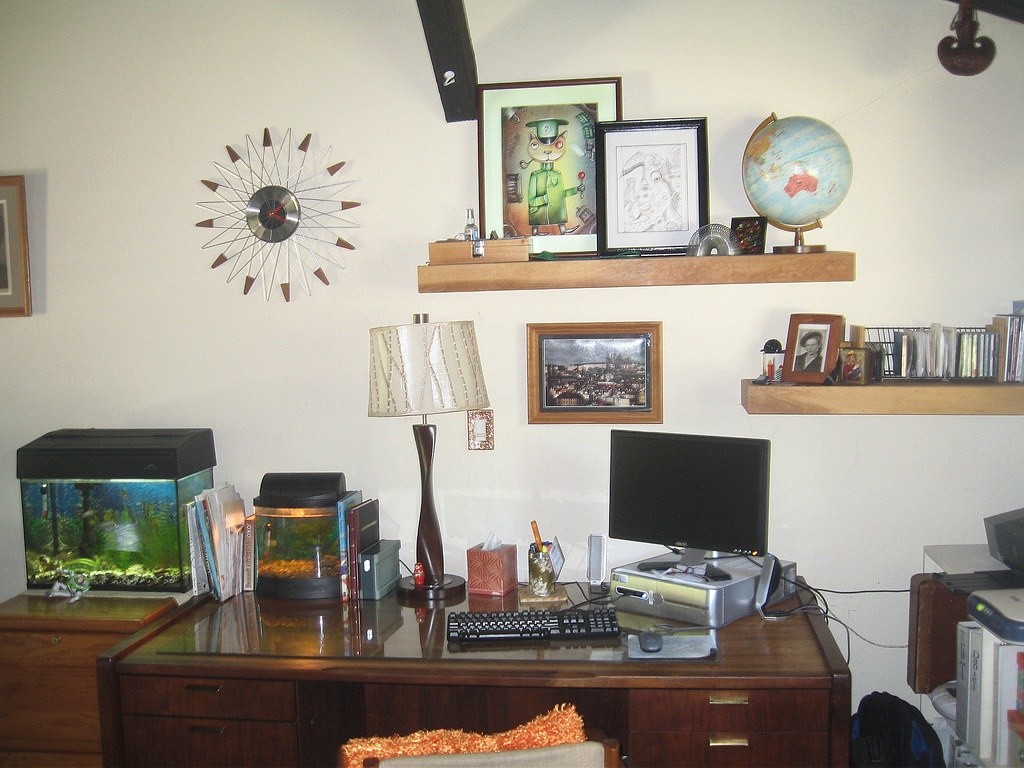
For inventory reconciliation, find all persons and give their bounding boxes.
[843,350,861,382]
[794,331,823,372]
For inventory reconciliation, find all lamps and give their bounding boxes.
[367,312,491,602]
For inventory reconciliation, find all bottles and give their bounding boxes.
[464,208,479,241]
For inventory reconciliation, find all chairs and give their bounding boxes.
[358,736,622,768]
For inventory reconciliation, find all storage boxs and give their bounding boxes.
[427,238,534,264]
[18,466,215,594]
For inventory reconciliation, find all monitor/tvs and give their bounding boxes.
[609,430,771,581]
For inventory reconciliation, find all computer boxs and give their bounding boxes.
[610,548,797,627]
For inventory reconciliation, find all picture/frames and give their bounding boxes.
[0,174,33,318]
[594,116,710,260]
[839,347,869,386]
[475,76,624,260]
[466,408,495,451]
[525,322,664,425]
[781,314,845,385]
[729,217,768,256]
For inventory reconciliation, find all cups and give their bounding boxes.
[529,541,555,597]
[763,353,784,382]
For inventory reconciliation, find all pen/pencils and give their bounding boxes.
[532,541,552,553]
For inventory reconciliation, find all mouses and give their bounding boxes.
[638,632,663,652]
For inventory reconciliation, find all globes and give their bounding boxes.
[743,112,853,254]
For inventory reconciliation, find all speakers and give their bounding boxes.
[587,533,606,586]
[755,554,790,620]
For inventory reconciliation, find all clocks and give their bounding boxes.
[195,124,362,303]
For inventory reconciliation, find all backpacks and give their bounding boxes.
[849,692,946,768]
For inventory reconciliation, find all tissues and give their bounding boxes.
[466,531,520,597]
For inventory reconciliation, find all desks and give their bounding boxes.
[94,575,853,768]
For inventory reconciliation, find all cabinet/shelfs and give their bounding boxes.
[0,592,180,768]
[418,248,1024,417]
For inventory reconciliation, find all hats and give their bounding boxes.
[847,350,856,357]
[800,331,823,347]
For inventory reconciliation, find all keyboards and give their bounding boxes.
[447,608,620,642]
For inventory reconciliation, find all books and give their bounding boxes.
[194,591,262,654]
[186,484,256,603]
[343,601,382,659]
[338,490,378,601]
[955,622,1024,766]
[866,301,1024,382]
